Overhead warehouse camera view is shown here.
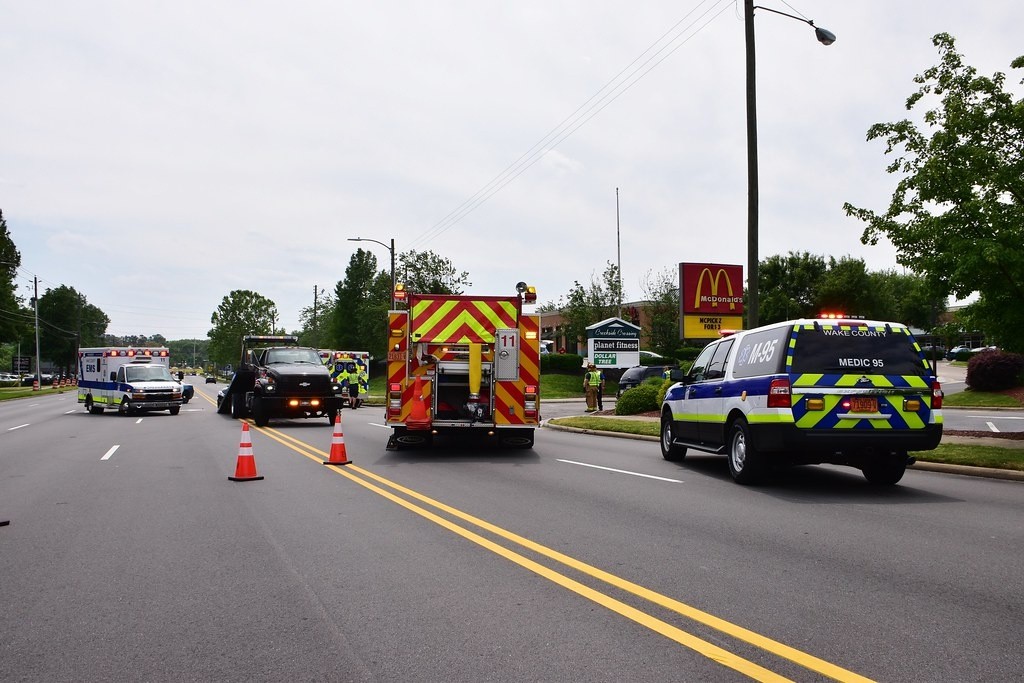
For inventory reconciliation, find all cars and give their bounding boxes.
[181,381,194,404]
[0,373,66,383]
[206,375,217,384]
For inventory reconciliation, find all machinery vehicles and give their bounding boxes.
[217,336,344,428]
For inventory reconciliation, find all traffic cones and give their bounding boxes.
[226,422,266,482]
[322,417,353,466]
[406,375,431,429]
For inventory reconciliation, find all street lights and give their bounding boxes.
[346,236,397,311]
[744,0,837,333]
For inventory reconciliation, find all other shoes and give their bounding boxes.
[352,407,356,409]
[585,409,597,413]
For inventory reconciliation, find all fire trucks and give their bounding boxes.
[384,281,543,451]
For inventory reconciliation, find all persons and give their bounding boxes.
[661,366,672,379]
[583,363,605,411]
[346,367,364,409]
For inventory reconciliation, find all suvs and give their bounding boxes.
[659,316,947,489]
[616,365,679,402]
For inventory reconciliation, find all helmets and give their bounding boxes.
[351,367,357,372]
[587,363,595,368]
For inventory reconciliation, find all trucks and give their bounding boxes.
[76,347,184,416]
[316,348,371,409]
